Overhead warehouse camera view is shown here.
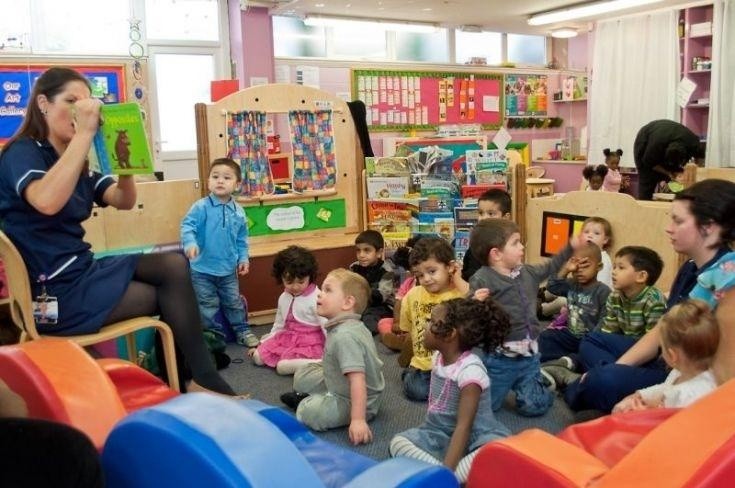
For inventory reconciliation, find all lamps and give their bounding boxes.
[526,0,663,28]
[552,27,578,38]
[303,14,440,33]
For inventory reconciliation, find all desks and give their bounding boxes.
[525,178,555,197]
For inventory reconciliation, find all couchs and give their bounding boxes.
[0,335,182,455]
[464,378,735,488]
[98,388,462,487]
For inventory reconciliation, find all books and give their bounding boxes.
[453,149,511,186]
[66,98,155,177]
[452,183,507,263]
[418,147,462,236]
[362,154,418,235]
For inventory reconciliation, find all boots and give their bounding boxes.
[542,364,580,386]
[538,355,574,369]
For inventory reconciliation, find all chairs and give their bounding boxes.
[0,226,181,396]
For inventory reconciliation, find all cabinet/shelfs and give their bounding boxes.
[678,3,715,168]
[360,162,530,266]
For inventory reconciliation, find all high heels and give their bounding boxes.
[182,377,251,403]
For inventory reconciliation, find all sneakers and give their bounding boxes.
[278,391,310,410]
[392,299,402,334]
[382,333,401,352]
[398,333,414,368]
[234,330,261,348]
[539,368,556,392]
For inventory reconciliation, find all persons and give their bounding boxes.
[538,242,611,362]
[397,236,471,404]
[460,186,515,280]
[386,291,516,484]
[179,156,261,347]
[376,235,422,368]
[465,216,586,418]
[347,228,396,335]
[246,241,329,378]
[557,247,671,370]
[534,215,616,322]
[278,266,388,448]
[601,148,632,192]
[609,299,721,416]
[543,178,735,416]
[632,118,705,200]
[581,163,609,192]
[1,66,253,404]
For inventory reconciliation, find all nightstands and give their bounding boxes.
[533,164,586,194]
